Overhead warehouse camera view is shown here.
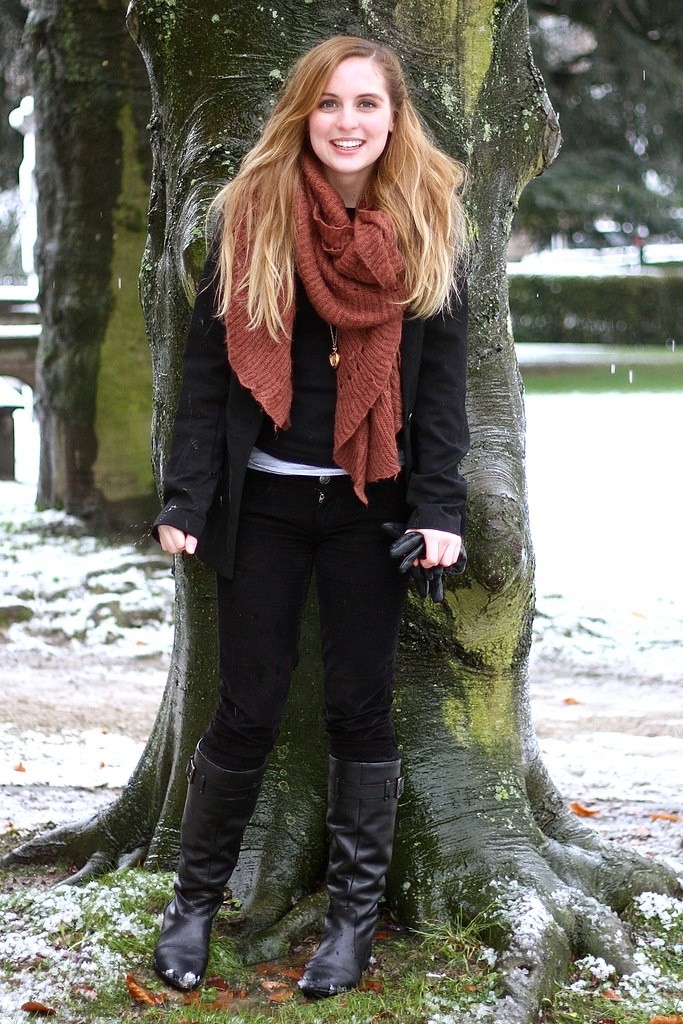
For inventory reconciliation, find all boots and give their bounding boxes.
[153,738,272,991]
[298,754,403,997]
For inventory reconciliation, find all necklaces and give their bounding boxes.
[328,323,340,370]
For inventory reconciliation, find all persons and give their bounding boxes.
[151,36,470,995]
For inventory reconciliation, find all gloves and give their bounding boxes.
[390,532,469,603]
